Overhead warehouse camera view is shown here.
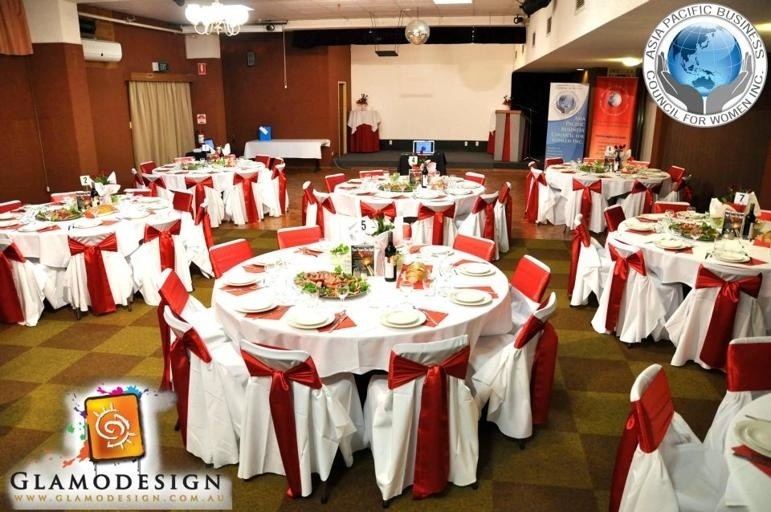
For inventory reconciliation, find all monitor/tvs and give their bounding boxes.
[413,140,435,156]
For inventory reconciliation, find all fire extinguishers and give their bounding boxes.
[194,128,205,148]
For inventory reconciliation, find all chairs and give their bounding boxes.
[0,136,771,512]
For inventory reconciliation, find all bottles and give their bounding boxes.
[422,162,429,188]
[385,230,397,282]
[743,202,757,241]
[614,152,620,173]
[219,144,224,159]
[91,182,100,207]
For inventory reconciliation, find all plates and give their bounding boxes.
[224,238,336,329]
[552,163,668,181]
[729,417,771,459]
[383,245,498,330]
[340,177,482,201]
[156,162,259,176]
[624,211,750,264]
[0,195,170,228]
[746,420,771,452]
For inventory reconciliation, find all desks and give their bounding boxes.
[342,108,384,155]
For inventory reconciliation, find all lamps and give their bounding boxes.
[403,5,432,46]
[180,1,256,40]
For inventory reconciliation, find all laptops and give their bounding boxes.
[204,137,219,153]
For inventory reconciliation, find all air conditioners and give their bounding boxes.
[79,34,126,65]
